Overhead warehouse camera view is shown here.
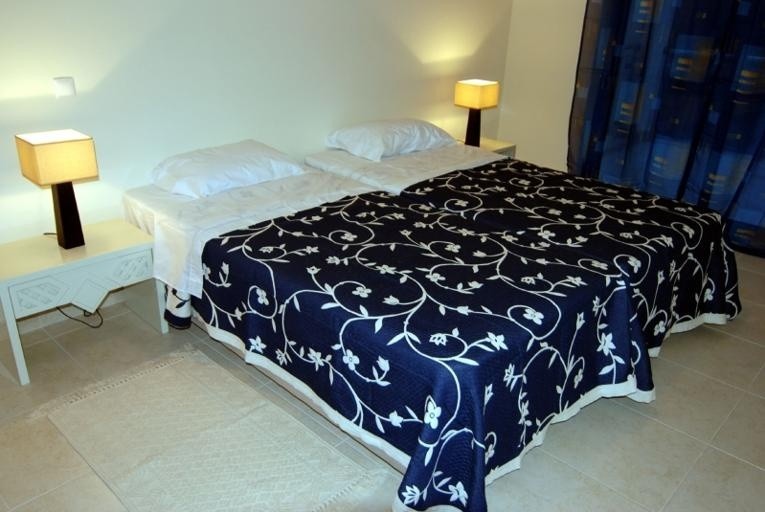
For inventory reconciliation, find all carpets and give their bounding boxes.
[37,343,379,512]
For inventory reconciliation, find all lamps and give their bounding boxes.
[454,78,500,147]
[15,130,99,249]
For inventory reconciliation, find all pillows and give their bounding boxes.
[151,141,311,197]
[326,118,453,162]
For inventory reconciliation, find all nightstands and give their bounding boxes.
[0,219,169,385]
[457,137,516,157]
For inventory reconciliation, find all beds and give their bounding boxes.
[123,118,741,512]
